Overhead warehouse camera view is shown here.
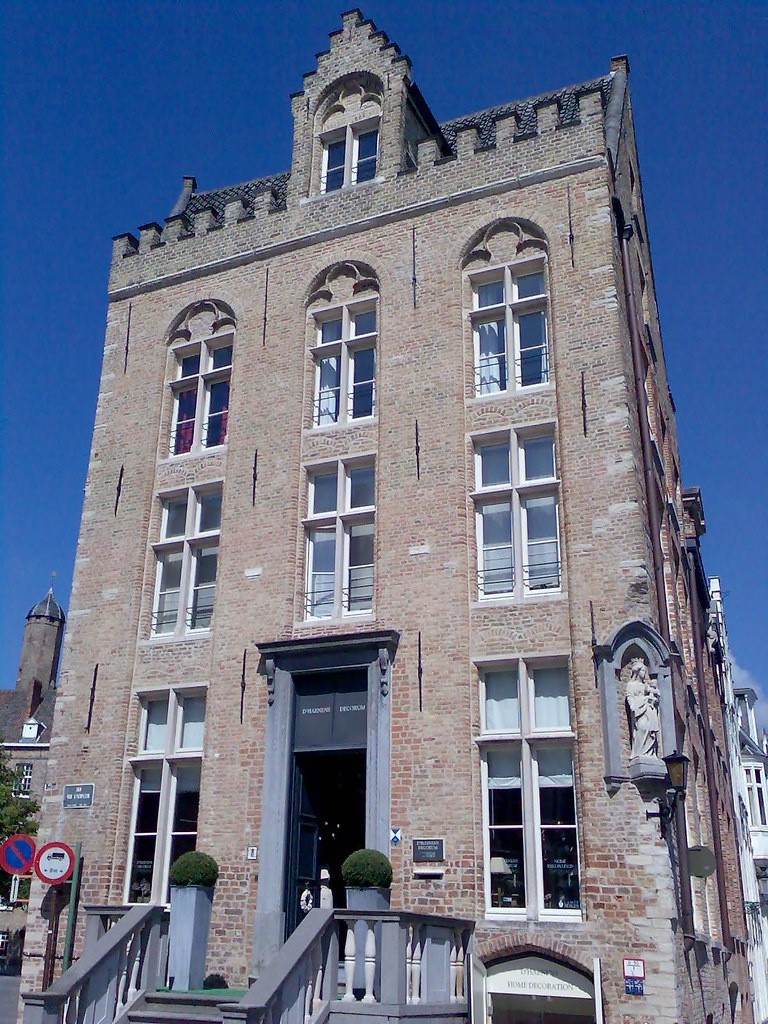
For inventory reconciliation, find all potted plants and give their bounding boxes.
[166,850,219,989]
[342,848,393,1002]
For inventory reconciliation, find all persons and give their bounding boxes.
[644,679,660,708]
[627,661,660,759]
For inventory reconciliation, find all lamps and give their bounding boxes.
[490,858,512,908]
[662,749,696,951]
